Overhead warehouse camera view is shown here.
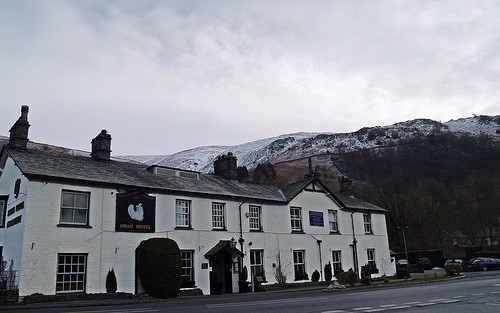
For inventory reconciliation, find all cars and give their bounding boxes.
[397,259,408,268]
[417,258,432,270]
[444,256,500,272]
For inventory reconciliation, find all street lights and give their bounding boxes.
[396,226,408,260]
